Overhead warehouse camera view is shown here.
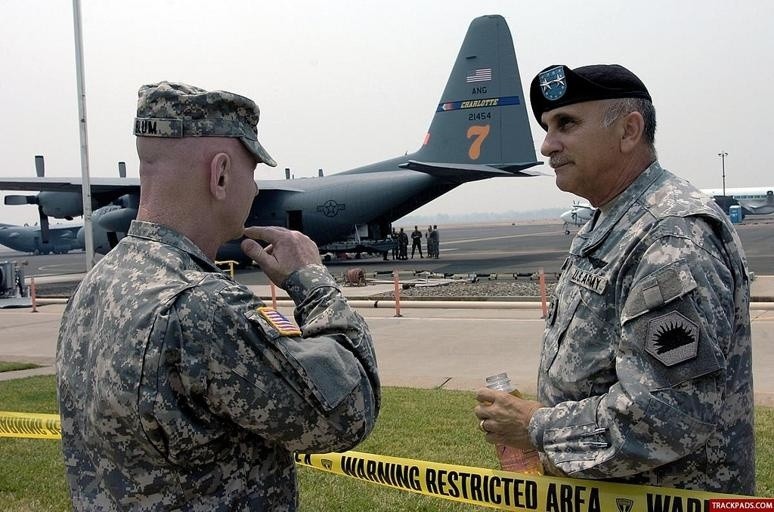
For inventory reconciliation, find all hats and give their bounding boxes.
[530,64,652,128]
[134,81,277,167]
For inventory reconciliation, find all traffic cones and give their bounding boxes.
[271,282,277,310]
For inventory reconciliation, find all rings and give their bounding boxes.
[478,418,487,433]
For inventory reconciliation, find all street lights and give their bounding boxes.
[718,150,728,196]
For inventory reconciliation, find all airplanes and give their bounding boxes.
[1,13,545,268]
[559,187,773,235]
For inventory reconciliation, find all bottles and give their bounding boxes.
[485,372,544,477]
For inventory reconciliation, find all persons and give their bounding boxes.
[51,78,383,510]
[473,60,757,512]
[390,222,440,261]
